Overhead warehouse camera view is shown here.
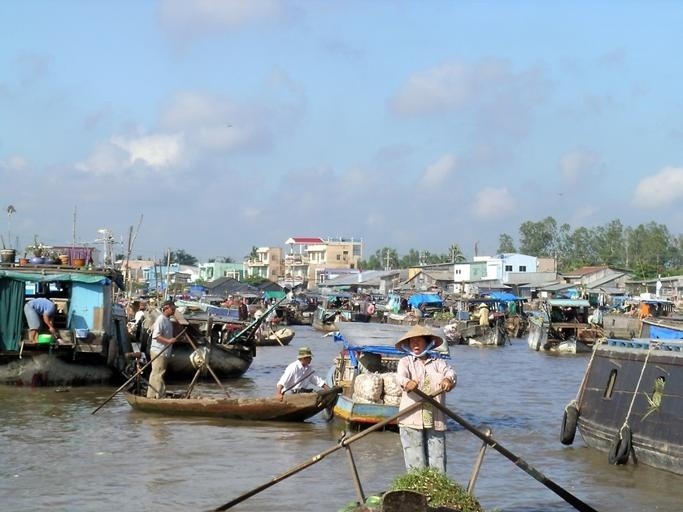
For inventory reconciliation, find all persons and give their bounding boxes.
[133,304,145,325]
[398,298,408,314]
[24,298,64,344]
[395,325,457,477]
[473,303,489,330]
[176,286,375,333]
[276,346,330,401]
[147,300,177,400]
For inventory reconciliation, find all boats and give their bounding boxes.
[332,469,485,512]
[126,313,258,381]
[560,338,683,477]
[0,264,120,389]
[324,321,451,426]
[122,382,342,422]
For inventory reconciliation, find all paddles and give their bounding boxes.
[414,388,599,512]
[206,386,447,512]
[92,327,186,415]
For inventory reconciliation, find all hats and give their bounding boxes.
[395,323,443,351]
[55,301,68,314]
[480,303,487,307]
[161,300,178,309]
[297,346,314,359]
